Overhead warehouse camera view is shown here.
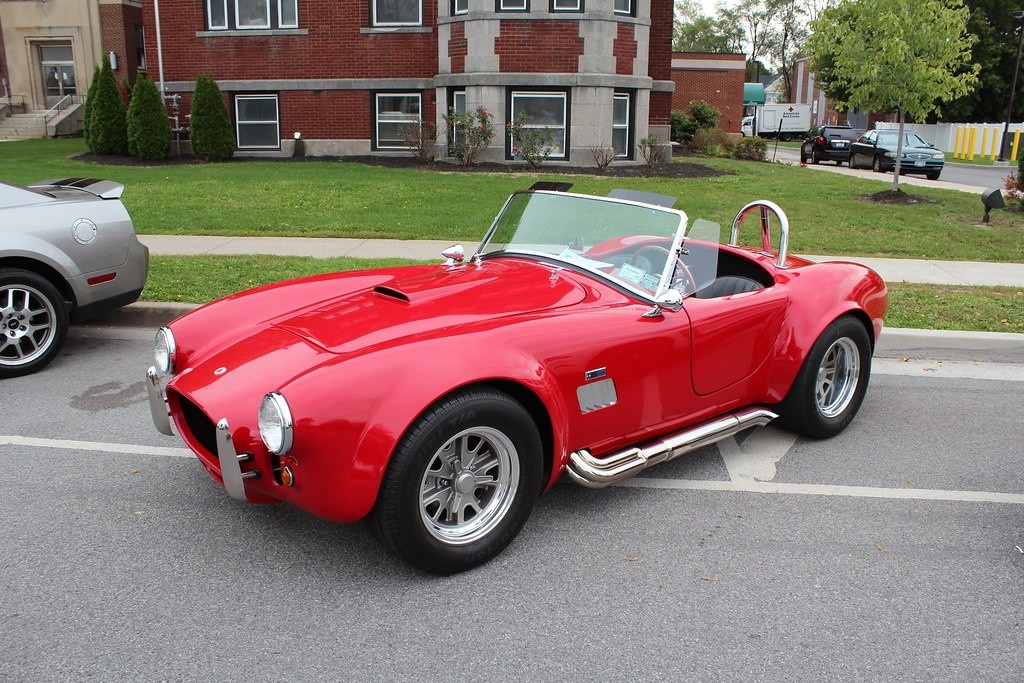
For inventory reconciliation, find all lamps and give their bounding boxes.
[981,188,1006,209]
[110,51,117,69]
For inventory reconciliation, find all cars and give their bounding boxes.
[850,129,944,180]
[0,178,149,380]
[146,190,887,576]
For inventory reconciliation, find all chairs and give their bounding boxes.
[697,275,763,298]
[605,254,651,274]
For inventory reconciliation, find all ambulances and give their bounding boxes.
[742,105,813,141]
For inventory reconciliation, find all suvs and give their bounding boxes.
[801,125,859,164]
